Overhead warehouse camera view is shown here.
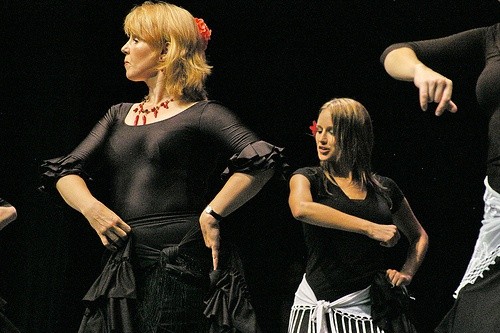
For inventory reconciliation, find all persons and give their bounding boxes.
[286,97,429,333]
[379,22,500,333]
[0,196,18,230]
[38,0,289,333]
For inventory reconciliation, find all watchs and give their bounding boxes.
[203,204,224,222]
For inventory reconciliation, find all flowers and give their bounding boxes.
[310,120,317,138]
[193,16,213,51]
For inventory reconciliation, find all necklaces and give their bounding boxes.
[133,94,179,127]
